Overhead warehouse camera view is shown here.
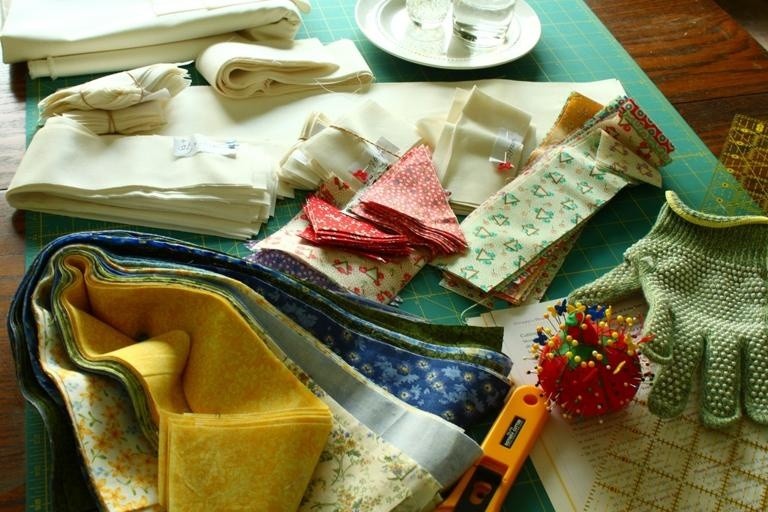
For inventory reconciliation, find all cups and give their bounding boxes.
[452,0,516,50]
[404,1,450,32]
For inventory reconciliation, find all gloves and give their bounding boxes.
[568,189,764,431]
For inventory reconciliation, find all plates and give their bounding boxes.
[353,1,541,72]
